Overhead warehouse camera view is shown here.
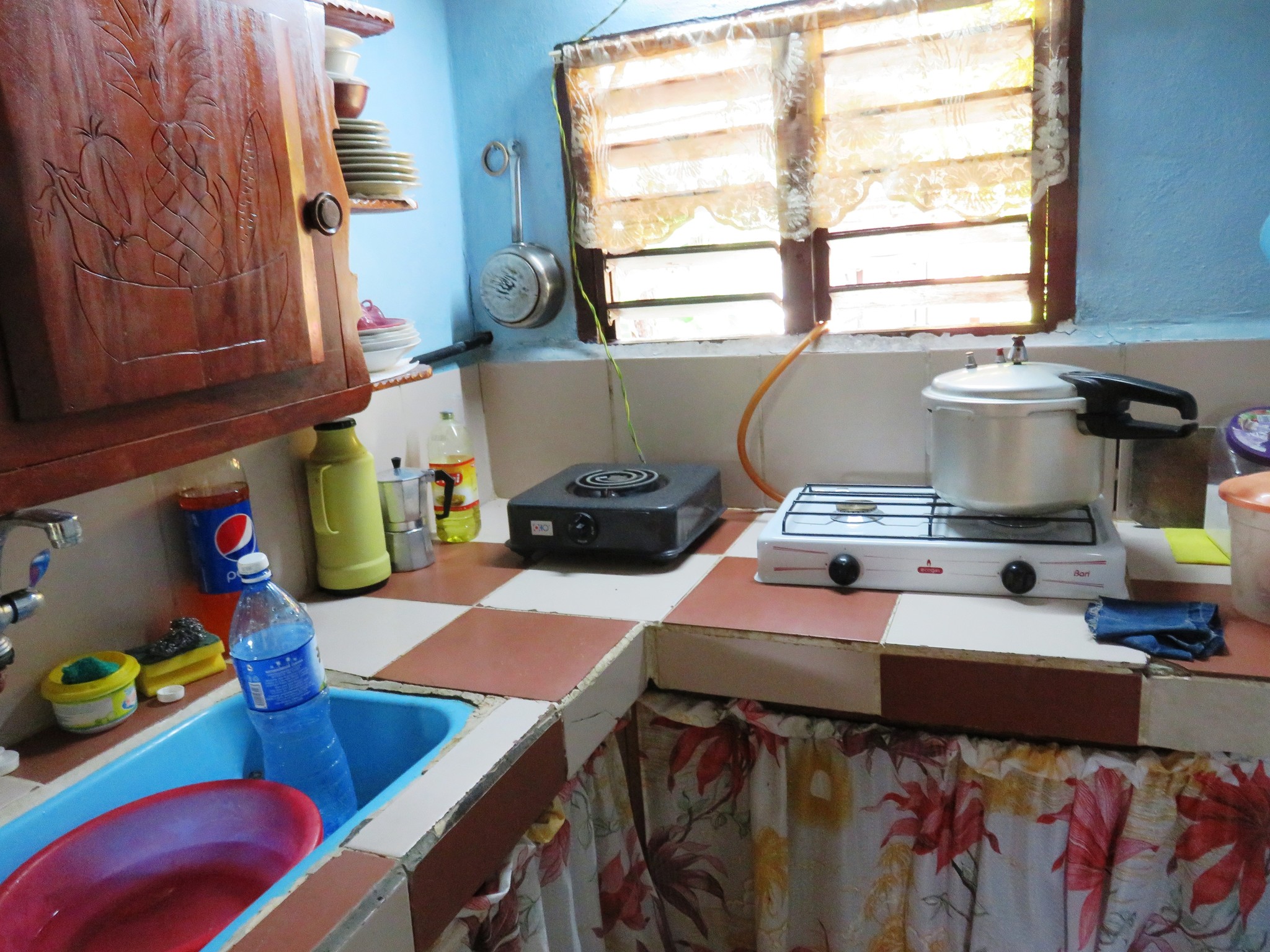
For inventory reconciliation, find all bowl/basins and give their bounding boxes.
[40,652,139,737]
[1216,472,1270,626]
[324,24,369,119]
[0,778,319,951]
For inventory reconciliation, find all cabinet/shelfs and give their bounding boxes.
[0,0,433,518]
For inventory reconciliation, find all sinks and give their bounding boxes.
[0,687,475,951]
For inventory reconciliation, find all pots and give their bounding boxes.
[921,336,1198,517]
[479,142,566,328]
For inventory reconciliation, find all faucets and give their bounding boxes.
[0,505,80,669]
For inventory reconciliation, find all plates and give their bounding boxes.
[357,317,421,373]
[332,117,422,195]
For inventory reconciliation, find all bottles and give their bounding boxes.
[306,419,391,592]
[230,555,358,837]
[177,450,261,656]
[429,413,482,541]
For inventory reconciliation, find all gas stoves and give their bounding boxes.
[505,463,727,568]
[753,486,1127,601]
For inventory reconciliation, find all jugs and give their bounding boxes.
[376,457,455,570]
